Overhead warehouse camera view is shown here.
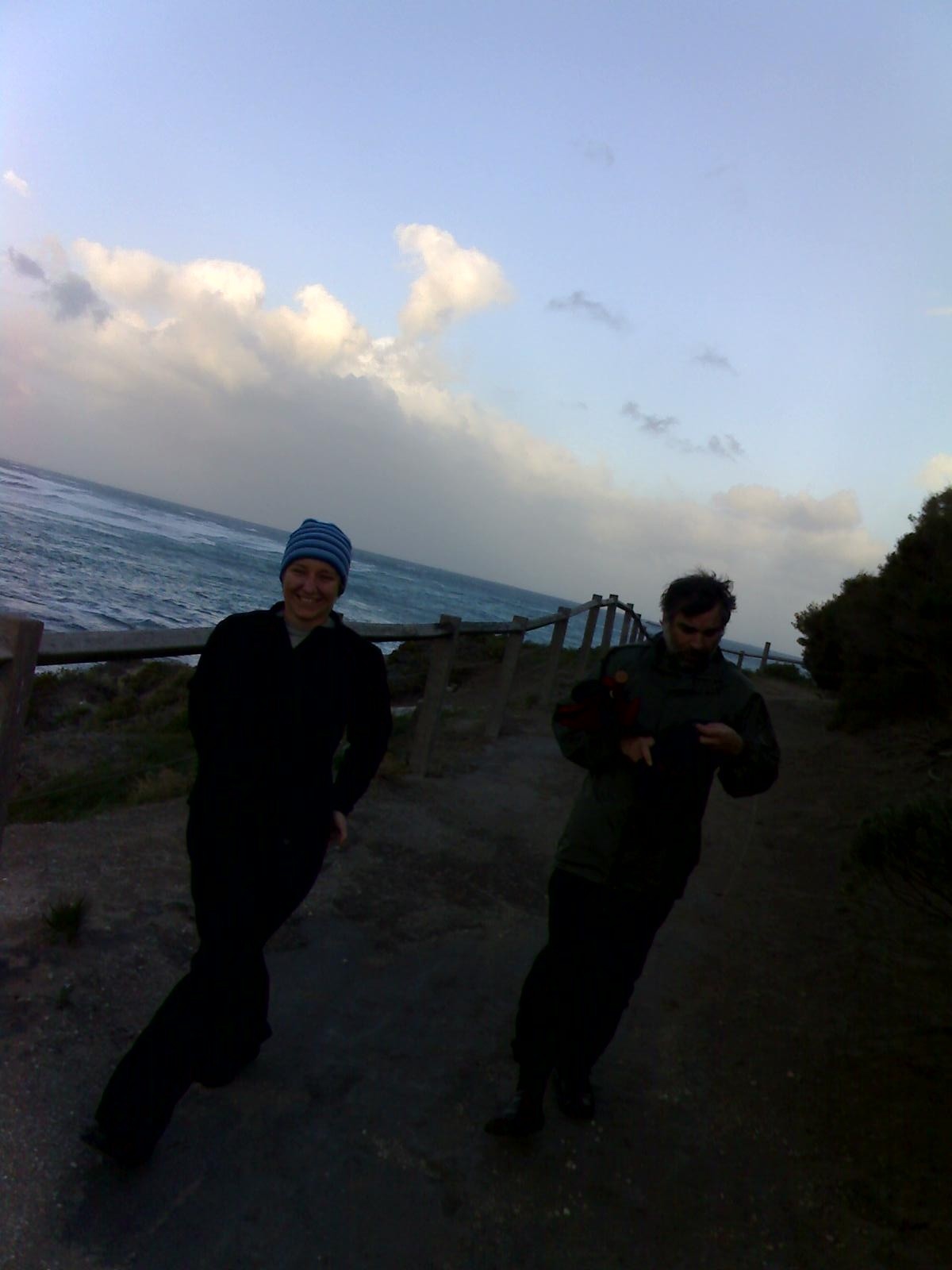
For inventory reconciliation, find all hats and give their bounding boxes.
[279,519,351,596]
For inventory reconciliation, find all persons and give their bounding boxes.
[480,574,779,1136]
[77,518,396,1172]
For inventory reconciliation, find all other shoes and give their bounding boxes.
[554,1067,596,1120]
[487,1090,545,1138]
[185,1044,261,1087]
[82,1120,160,1166]
